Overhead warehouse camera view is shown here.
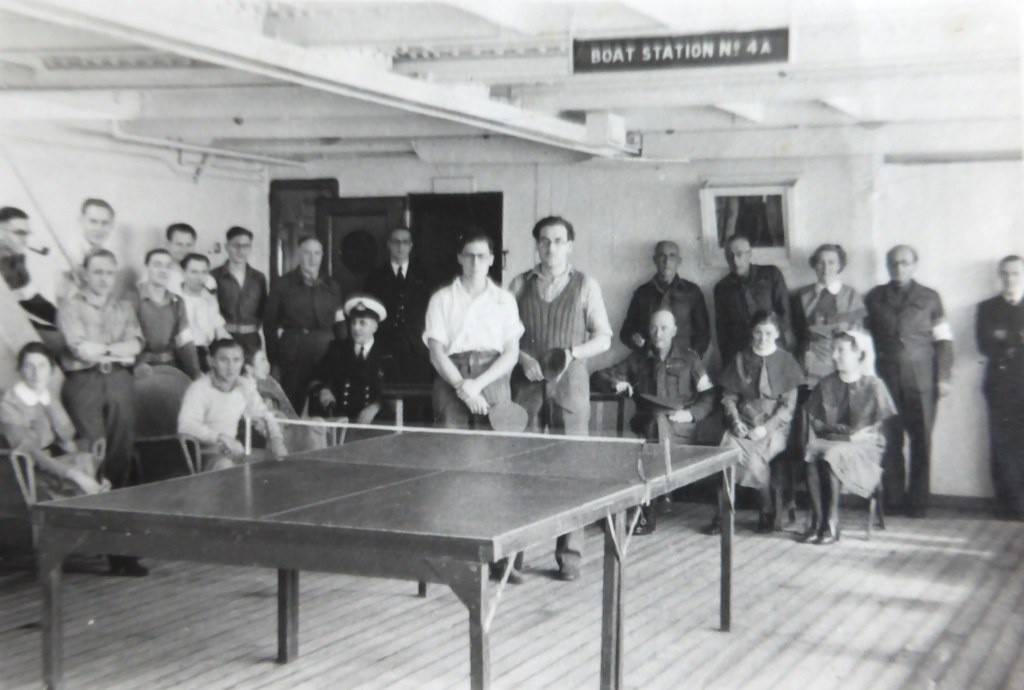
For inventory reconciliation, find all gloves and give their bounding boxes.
[817,422,852,442]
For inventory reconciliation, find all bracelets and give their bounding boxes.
[568,346,577,360]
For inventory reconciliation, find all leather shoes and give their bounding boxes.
[704,524,722,535]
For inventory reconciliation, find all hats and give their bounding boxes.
[488,401,528,433]
[842,329,876,376]
[640,392,684,414]
[344,296,387,323]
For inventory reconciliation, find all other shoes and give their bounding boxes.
[558,563,580,580]
[757,509,777,534]
[882,506,927,518]
[491,562,528,583]
[634,512,656,535]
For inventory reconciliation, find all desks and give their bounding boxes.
[378,379,629,438]
[34,446,745,690]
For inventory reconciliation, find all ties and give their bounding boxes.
[397,266,404,281]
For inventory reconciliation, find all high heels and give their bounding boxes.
[804,519,840,545]
[108,559,149,577]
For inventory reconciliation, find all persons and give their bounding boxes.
[302,296,405,430]
[975,256,1024,522]
[705,308,810,535]
[620,240,954,517]
[0,199,432,577]
[590,308,718,535]
[508,215,614,582]
[176,338,289,473]
[796,326,899,544]
[420,233,529,586]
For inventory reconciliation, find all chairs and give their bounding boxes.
[0,366,350,560]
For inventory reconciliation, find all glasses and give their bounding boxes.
[539,238,566,247]
[890,260,911,268]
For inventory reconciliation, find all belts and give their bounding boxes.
[82,362,125,374]
